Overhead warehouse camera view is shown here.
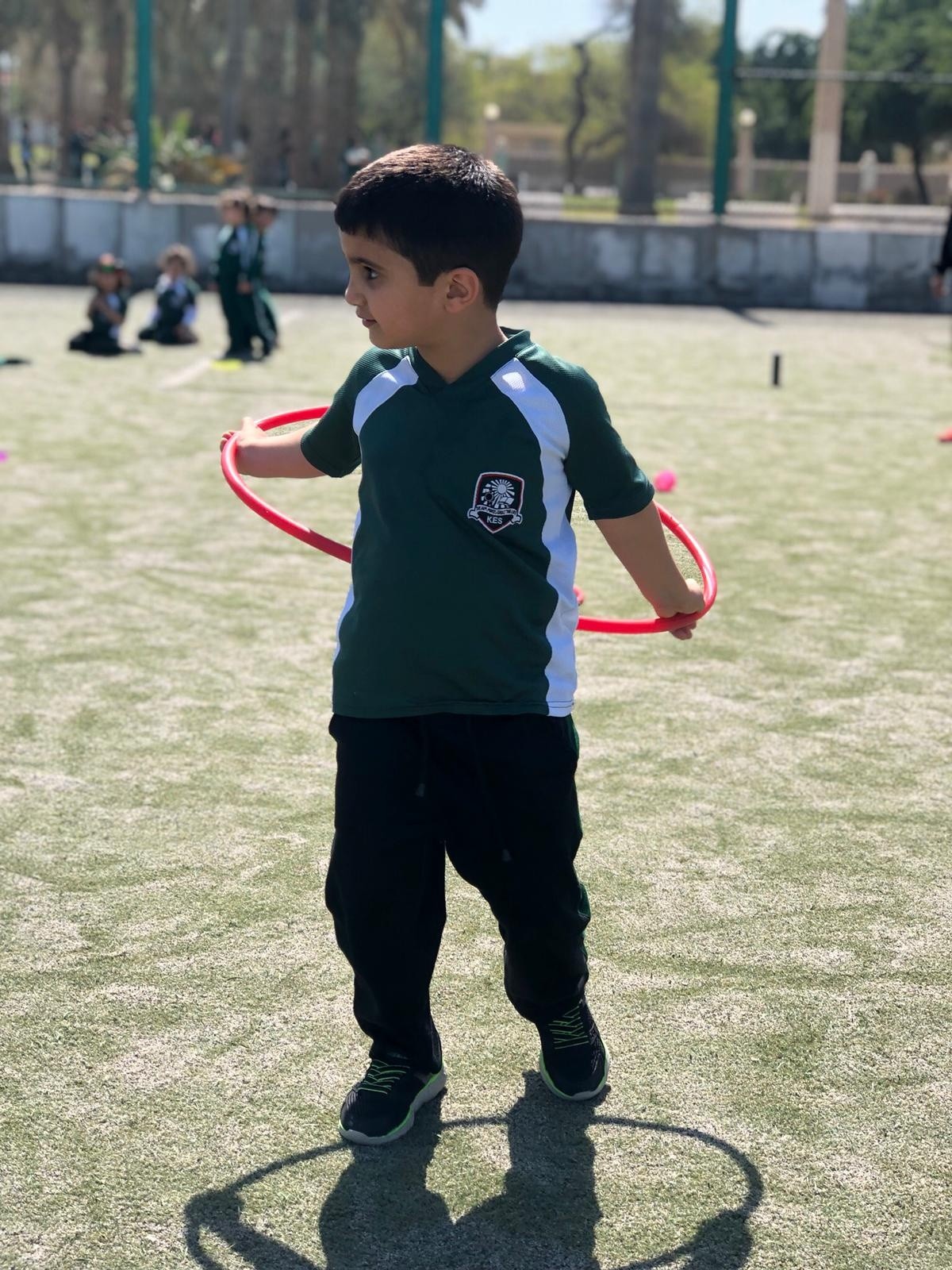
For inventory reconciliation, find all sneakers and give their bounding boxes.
[338,1056,447,1150]
[534,992,610,1102]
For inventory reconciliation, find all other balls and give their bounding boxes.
[652,469,676,493]
[940,428,952,441]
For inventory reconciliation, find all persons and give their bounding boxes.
[70,252,142,354]
[930,210,952,441]
[218,143,705,1145]
[137,243,200,346]
[216,194,279,360]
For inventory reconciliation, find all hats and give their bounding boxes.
[156,243,198,277]
